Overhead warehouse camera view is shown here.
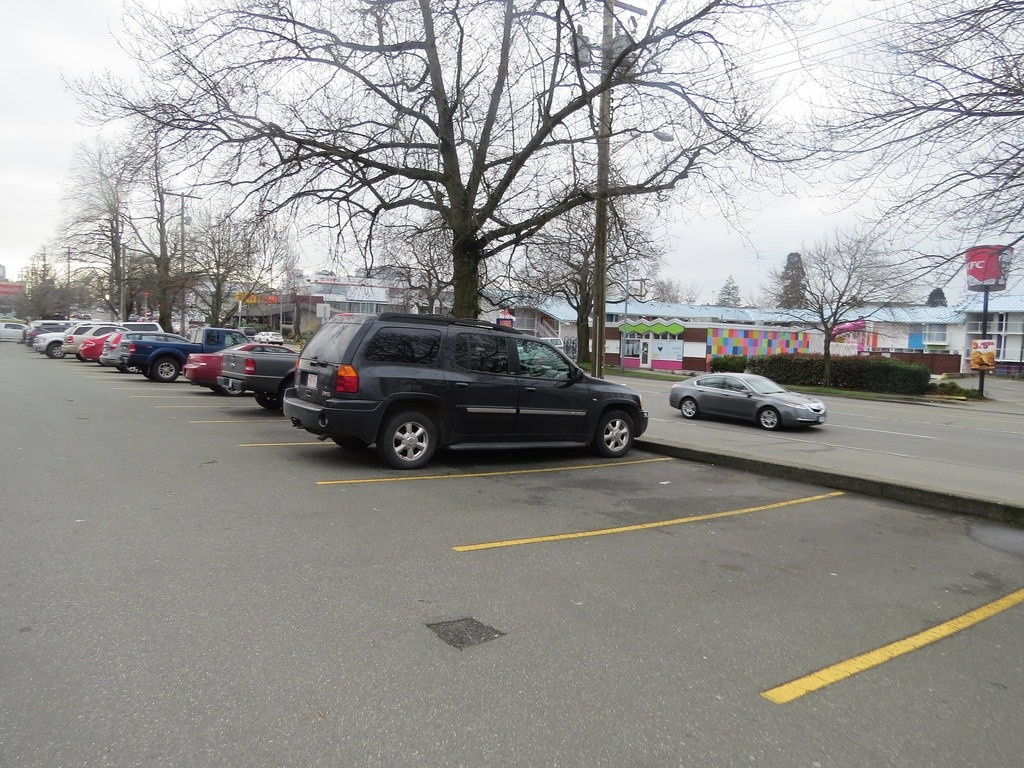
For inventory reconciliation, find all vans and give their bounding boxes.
[238,326,257,342]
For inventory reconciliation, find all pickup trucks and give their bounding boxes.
[216,349,300,413]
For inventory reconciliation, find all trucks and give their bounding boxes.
[126,327,250,384]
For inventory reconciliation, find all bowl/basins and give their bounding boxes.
[965,245,1014,290]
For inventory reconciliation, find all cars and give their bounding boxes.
[669,372,827,431]
[24,318,166,363]
[182,341,296,398]
[254,332,284,345]
[0,322,31,342]
[99,330,191,374]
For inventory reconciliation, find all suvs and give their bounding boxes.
[281,311,650,468]
